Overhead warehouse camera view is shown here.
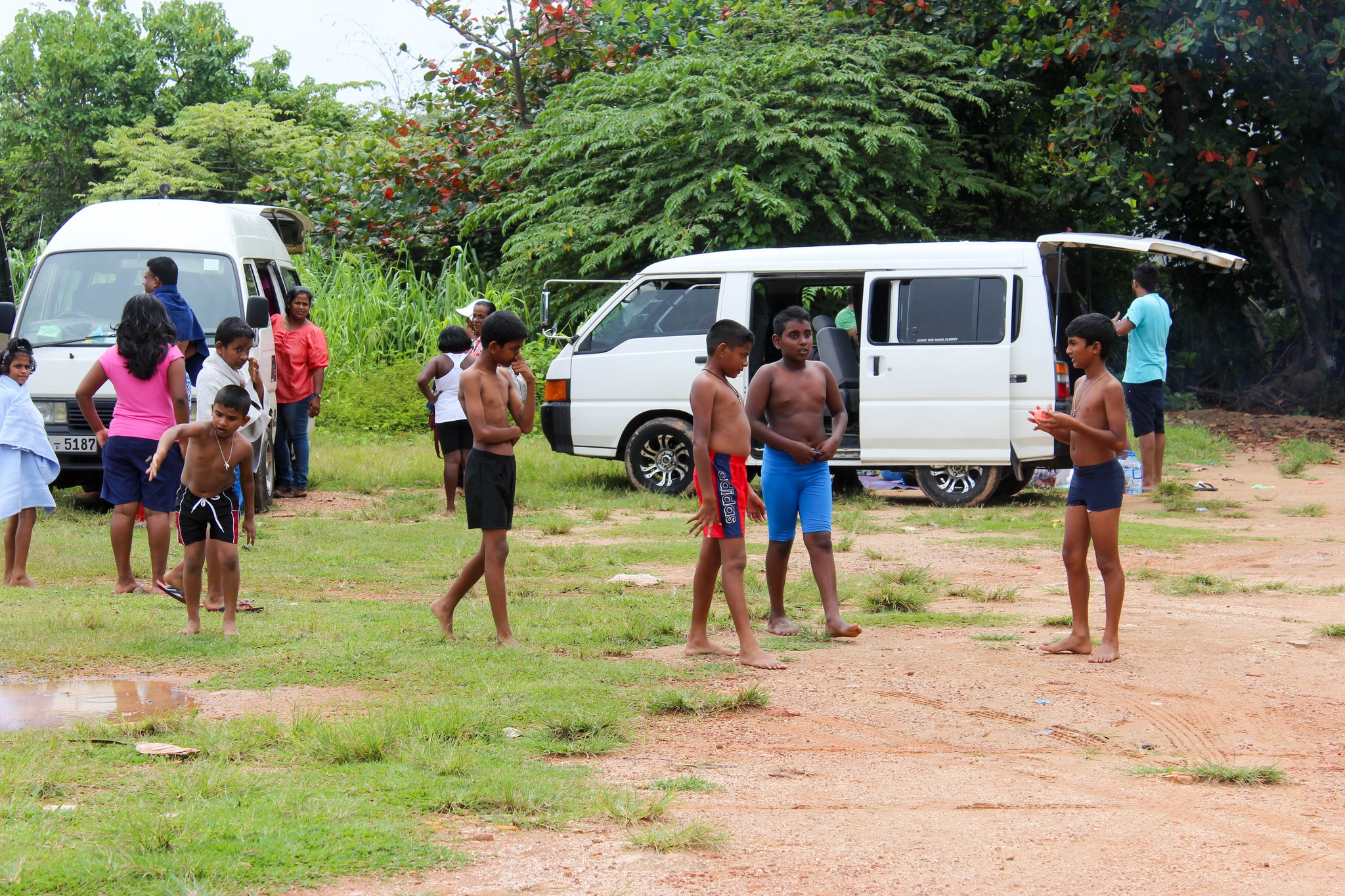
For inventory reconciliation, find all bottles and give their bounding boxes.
[1117,456,1124,466]
[1122,450,1143,494]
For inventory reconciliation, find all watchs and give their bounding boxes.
[314,393,321,398]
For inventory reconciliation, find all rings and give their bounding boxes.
[316,411,318,413]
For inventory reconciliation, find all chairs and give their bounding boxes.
[177,272,237,329]
[812,315,860,417]
[748,292,769,387]
[694,286,720,334]
[85,269,144,322]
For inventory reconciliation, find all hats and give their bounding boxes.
[456,299,491,322]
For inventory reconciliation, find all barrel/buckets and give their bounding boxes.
[135,499,146,522]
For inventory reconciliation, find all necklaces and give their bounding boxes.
[1071,371,1110,418]
[701,369,742,404]
[215,429,235,470]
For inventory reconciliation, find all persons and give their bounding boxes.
[832,285,858,342]
[142,257,211,417]
[1026,312,1127,663]
[74,292,189,594]
[1,338,61,589]
[156,315,270,614]
[745,307,862,639]
[880,470,903,482]
[430,311,536,651]
[143,383,256,637]
[426,298,527,502]
[680,318,789,670]
[269,285,330,497]
[417,325,478,515]
[1109,265,1173,490]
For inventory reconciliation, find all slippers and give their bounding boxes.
[156,580,204,608]
[111,584,150,595]
[1198,482,1219,491]
[274,487,288,496]
[1178,484,1199,491]
[160,593,170,596]
[279,490,307,497]
[209,600,264,613]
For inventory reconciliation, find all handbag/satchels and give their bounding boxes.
[505,365,528,401]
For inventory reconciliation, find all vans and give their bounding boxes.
[0,197,315,509]
[537,231,1253,514]
